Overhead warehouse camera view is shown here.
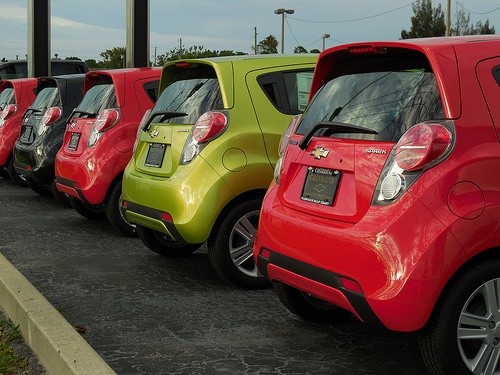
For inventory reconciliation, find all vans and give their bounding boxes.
[0,59,90,81]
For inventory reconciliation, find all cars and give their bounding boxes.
[12,73,85,209]
[52,67,163,240]
[0,77,38,189]
[252,32,500,375]
[119,51,320,294]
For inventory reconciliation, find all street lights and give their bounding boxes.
[275,8,294,54]
[252,26,258,55]
